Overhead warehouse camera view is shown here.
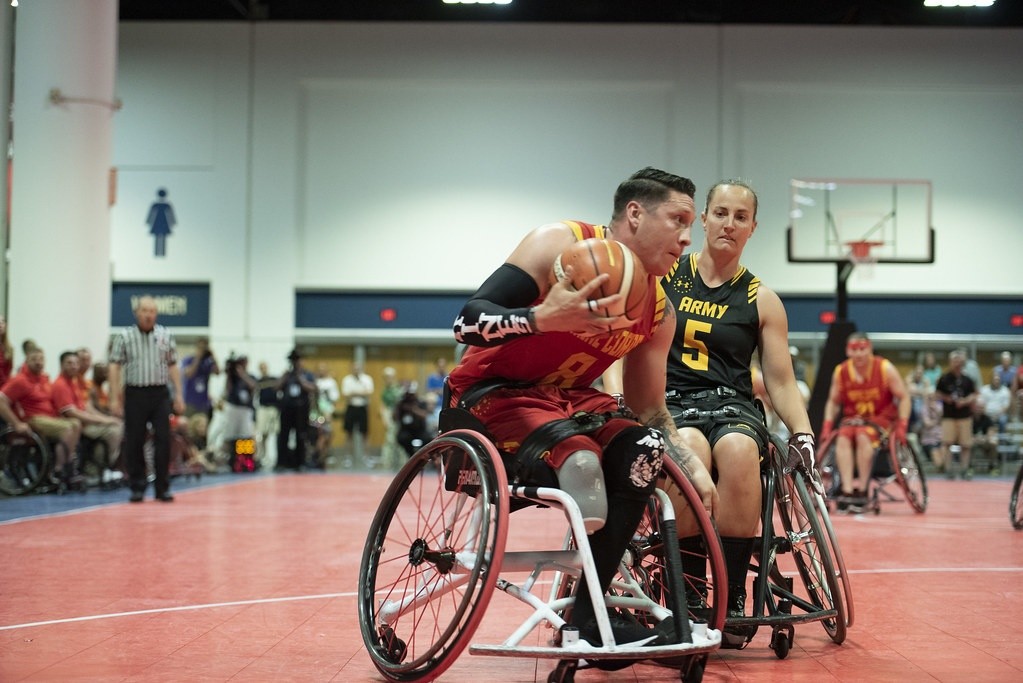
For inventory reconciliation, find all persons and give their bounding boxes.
[602,180,819,644]
[177,337,448,475]
[442,168,721,646]
[966,399,1001,476]
[906,347,1023,434]
[818,330,911,515]
[750,347,811,440]
[106,296,186,502]
[0,315,125,482]
[934,350,977,480]
[919,390,943,464]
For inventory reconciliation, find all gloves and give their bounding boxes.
[783,432,828,497]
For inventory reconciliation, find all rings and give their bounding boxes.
[589,300,600,312]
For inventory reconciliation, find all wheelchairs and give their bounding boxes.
[0,423,133,498]
[358,371,731,682]
[1008,463,1023,532]
[808,428,930,515]
[605,391,855,659]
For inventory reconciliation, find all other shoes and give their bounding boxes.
[130,490,143,501]
[156,492,173,501]
[849,488,868,513]
[836,492,853,512]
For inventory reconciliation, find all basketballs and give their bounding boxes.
[546,236,649,339]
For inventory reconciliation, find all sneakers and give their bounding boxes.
[726,581,746,617]
[686,577,707,609]
[578,614,660,649]
